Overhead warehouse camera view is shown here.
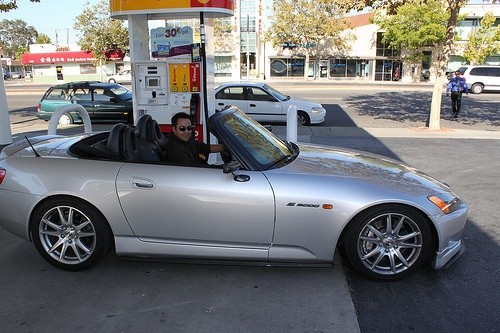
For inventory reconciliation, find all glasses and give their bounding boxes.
[456,74,461,75]
[178,126,193,132]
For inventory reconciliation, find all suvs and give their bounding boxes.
[453,66,500,94]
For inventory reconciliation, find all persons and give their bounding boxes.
[166,112,225,165]
[446,70,468,118]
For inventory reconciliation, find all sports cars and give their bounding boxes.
[0,104,467,281]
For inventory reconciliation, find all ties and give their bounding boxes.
[457,79,463,92]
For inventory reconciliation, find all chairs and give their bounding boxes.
[107,123,172,165]
[136,115,169,161]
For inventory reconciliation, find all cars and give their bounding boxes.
[3,71,32,80]
[321,68,327,75]
[214,82,327,127]
[36,80,132,125]
[330,64,354,75]
